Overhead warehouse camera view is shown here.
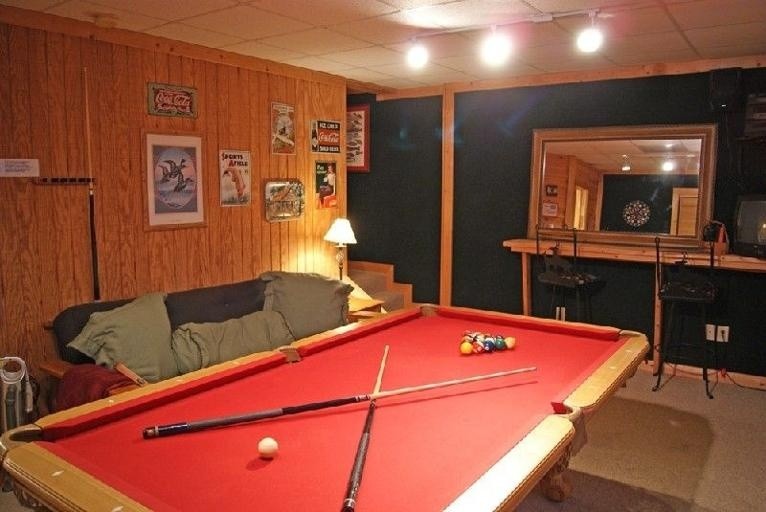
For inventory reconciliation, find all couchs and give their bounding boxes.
[38,271,387,396]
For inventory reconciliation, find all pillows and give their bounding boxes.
[65,268,355,384]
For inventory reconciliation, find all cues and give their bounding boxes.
[82,65,101,301]
[339,343,391,511]
[144,367,538,438]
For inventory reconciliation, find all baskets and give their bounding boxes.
[700,220,730,254]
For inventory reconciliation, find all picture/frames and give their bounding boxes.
[347,103,370,173]
[313,159,338,212]
[310,119,342,155]
[264,180,304,222]
[270,101,297,156]
[148,82,198,118]
[220,149,251,207]
[140,126,209,232]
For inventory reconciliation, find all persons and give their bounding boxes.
[317,163,335,209]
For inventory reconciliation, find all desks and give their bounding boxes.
[0,303,650,512]
[347,295,386,323]
[503,238,766,318]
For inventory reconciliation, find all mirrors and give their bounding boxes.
[527,123,719,250]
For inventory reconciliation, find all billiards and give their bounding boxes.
[460,330,516,353]
[256,437,277,458]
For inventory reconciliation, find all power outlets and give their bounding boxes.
[705,324,729,343]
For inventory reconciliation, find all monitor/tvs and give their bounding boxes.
[732,193,766,260]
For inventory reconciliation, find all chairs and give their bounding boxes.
[534,224,599,322]
[652,237,718,398]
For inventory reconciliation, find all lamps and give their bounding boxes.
[324,218,357,280]
